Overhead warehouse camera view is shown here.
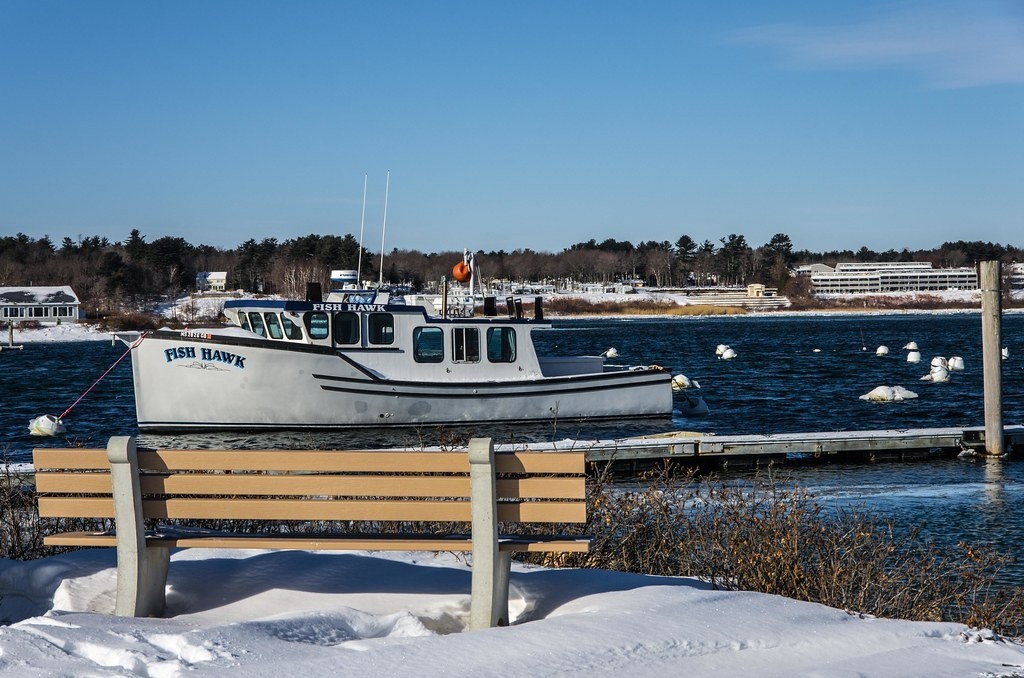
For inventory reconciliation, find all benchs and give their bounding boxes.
[33,436,590,632]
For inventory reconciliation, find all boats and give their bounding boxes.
[105,168,678,434]
